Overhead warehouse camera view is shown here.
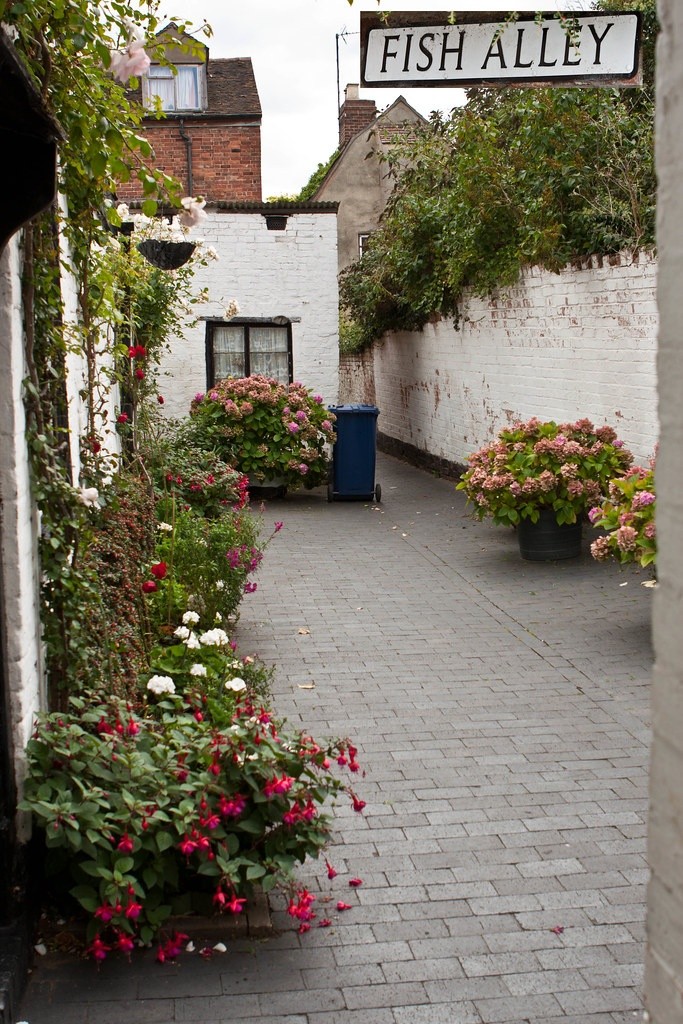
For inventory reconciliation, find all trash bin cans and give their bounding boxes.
[328,403,382,503]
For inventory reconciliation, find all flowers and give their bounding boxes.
[189,373,338,490]
[459,409,658,574]
[103,191,240,324]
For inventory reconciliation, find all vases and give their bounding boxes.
[136,238,196,271]
[511,501,587,563]
[241,463,285,487]
[109,221,135,236]
